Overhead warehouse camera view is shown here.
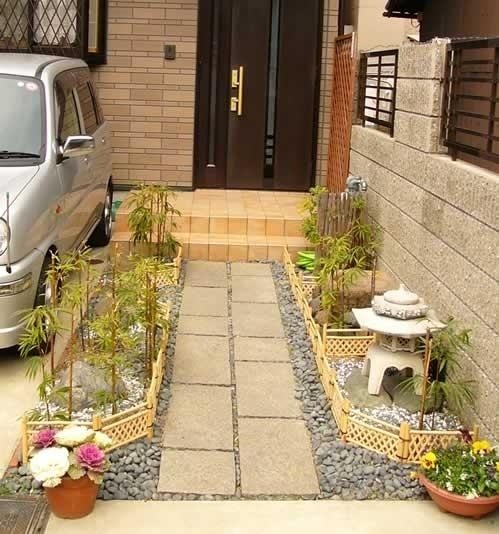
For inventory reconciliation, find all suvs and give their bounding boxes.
[0,53,115,355]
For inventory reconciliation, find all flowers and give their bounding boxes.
[416,440,498,500]
[27,425,117,491]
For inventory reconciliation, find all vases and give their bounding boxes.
[419,472,498,519]
[41,474,100,521]
